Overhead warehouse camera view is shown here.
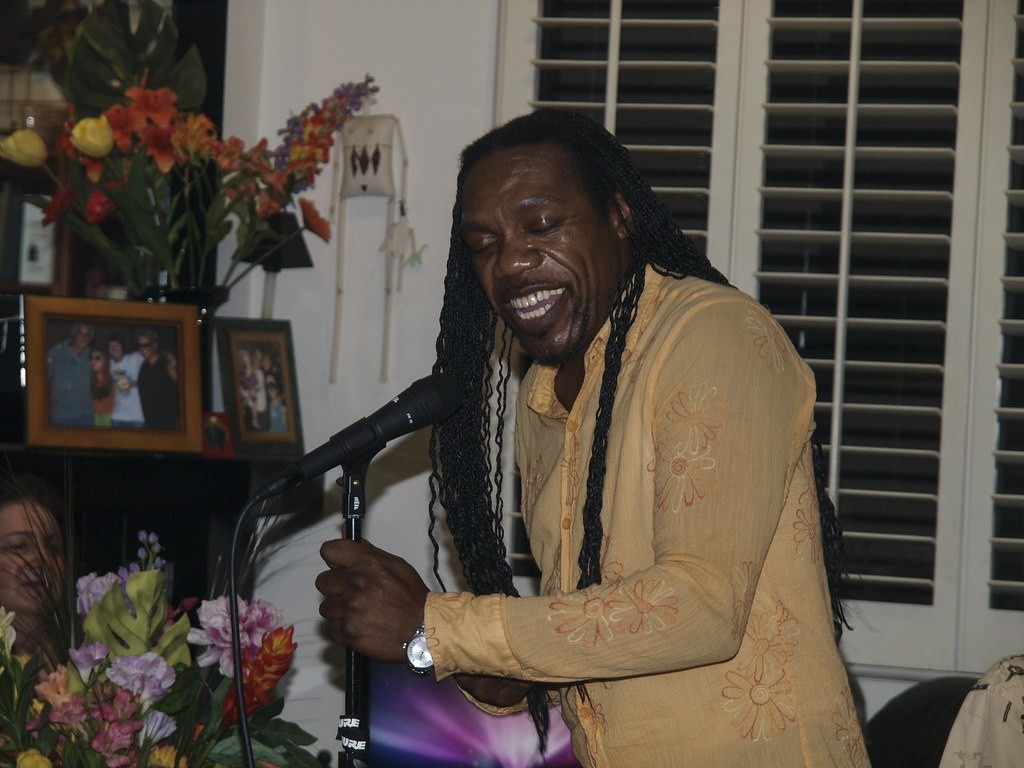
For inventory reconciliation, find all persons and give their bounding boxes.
[314,106,871,767]
[0,493,69,674]
[239,346,285,432]
[47,319,179,430]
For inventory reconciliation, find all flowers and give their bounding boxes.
[0,451,327,768]
[1,1,385,294]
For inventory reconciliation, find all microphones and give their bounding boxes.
[250,372,463,502]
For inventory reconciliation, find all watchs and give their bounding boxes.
[400,619,436,678]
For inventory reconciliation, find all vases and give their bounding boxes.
[136,285,229,410]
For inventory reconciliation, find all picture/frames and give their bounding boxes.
[214,318,305,456]
[18,294,204,456]
